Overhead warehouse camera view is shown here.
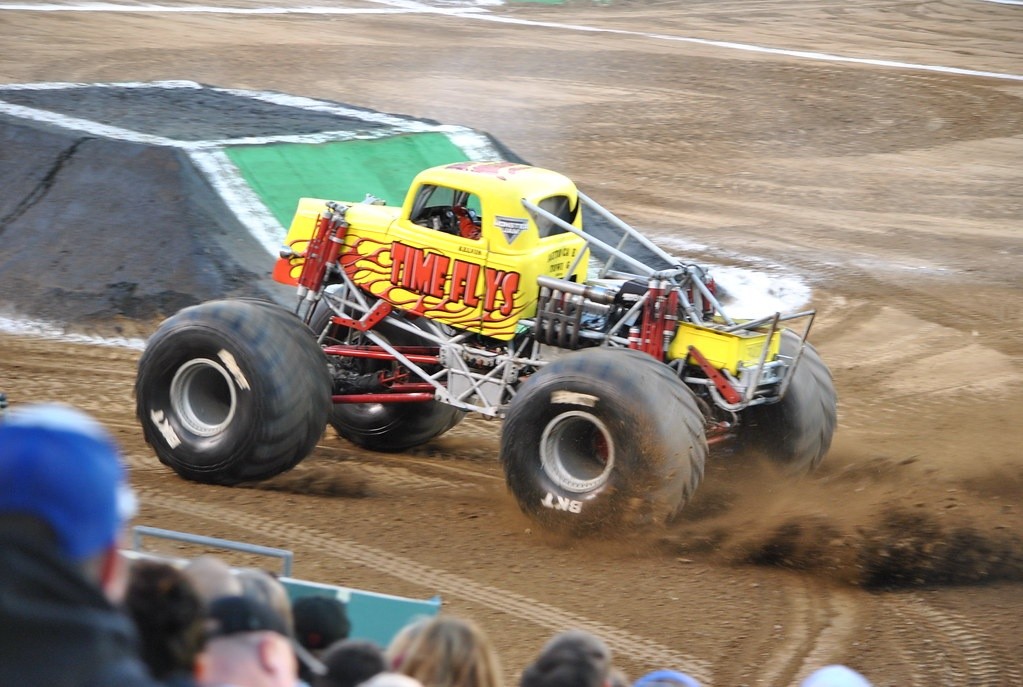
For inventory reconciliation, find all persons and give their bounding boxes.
[127,553,635,687]
[452,205,481,239]
[0,406,162,687]
[802,665,872,687]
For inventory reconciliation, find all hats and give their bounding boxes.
[634,670,699,687]
[0,404,138,563]
[293,595,350,649]
[204,594,326,675]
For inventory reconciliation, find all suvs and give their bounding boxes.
[136,159,839,556]
